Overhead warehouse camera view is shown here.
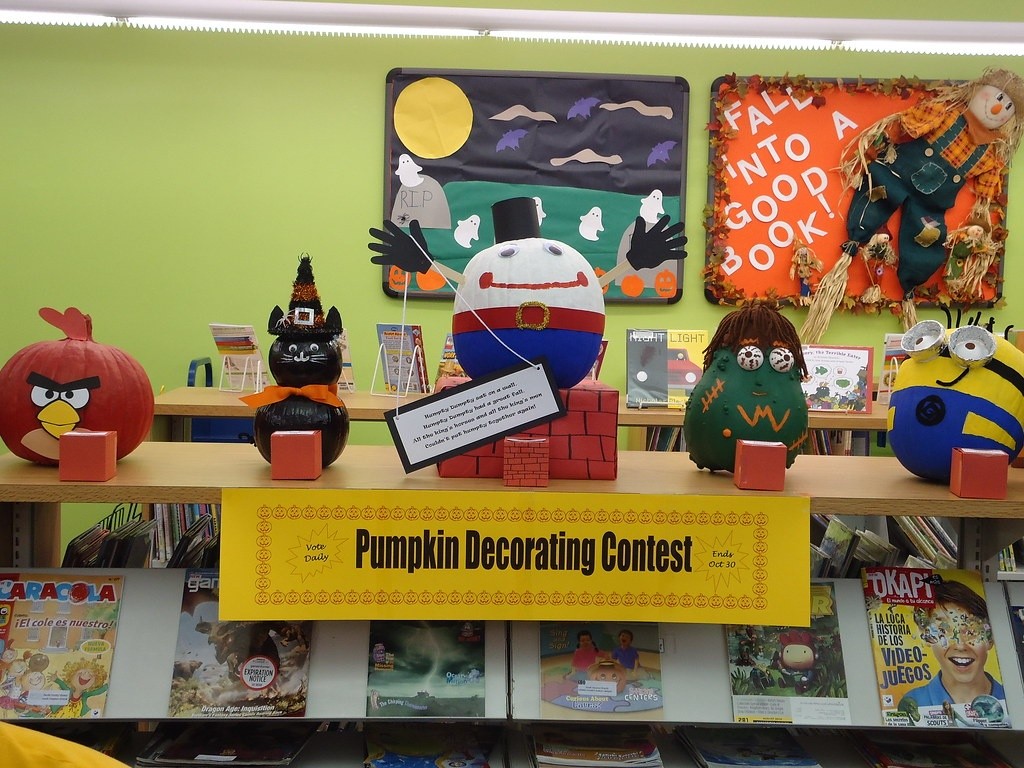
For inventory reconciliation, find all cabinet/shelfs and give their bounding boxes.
[0,441,1024,768]
[140,387,888,569]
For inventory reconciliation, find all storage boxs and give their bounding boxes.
[503,436,550,487]
[60,427,119,482]
[434,377,620,481]
[733,439,787,491]
[949,446,1009,499]
[271,430,322,481]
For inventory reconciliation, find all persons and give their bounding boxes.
[796,69,1024,344]
[939,698,975,728]
[944,220,991,282]
[897,579,1005,707]
[789,248,823,297]
[861,222,896,303]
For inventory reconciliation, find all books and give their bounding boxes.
[208,324,271,388]
[335,329,355,391]
[435,333,470,382]
[646,426,852,457]
[625,329,709,409]
[877,333,911,404]
[0,503,1024,768]
[376,324,431,394]
[799,345,874,415]
